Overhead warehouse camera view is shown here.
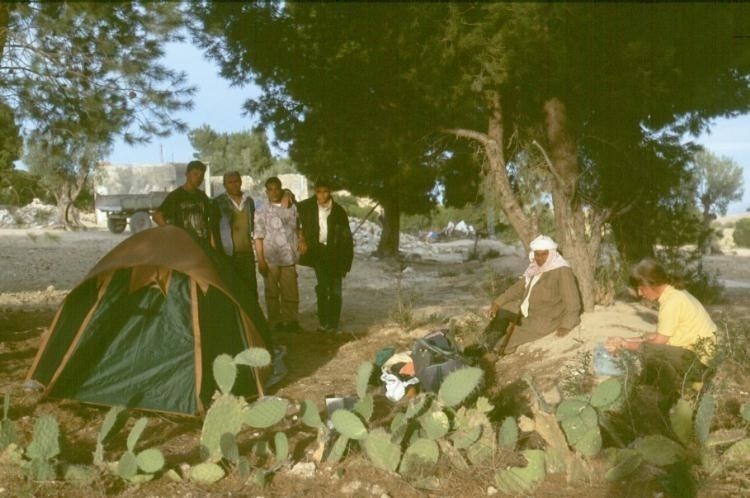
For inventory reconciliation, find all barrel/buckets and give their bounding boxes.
[589,344,628,393]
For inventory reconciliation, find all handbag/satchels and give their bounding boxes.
[412,328,465,392]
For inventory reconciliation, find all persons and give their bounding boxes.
[603,256,721,446]
[252,176,305,335]
[280,179,354,335]
[463,235,584,367]
[210,169,259,303]
[153,158,213,253]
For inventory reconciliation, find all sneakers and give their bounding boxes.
[317,325,342,336]
[286,324,302,332]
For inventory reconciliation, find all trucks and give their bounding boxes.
[93,188,172,236]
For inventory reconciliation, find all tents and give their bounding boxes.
[21,220,279,424]
